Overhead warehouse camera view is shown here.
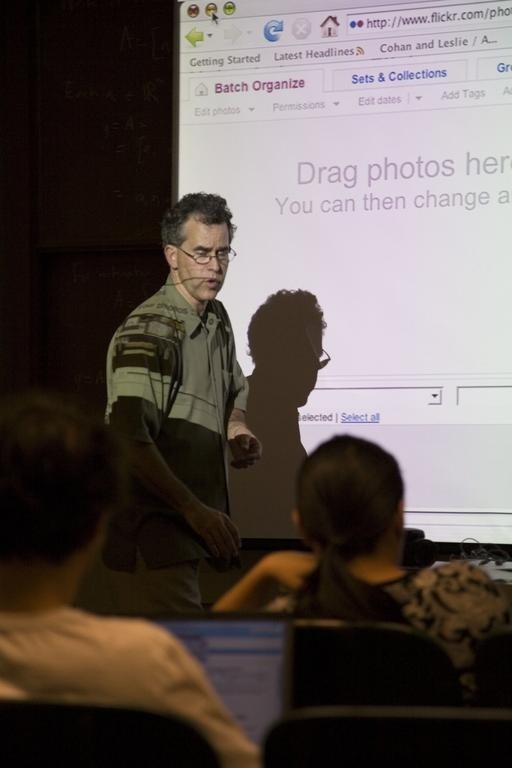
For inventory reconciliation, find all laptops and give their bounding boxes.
[128,614,293,745]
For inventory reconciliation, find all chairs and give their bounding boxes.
[1,701,229,766]
[259,706,510,766]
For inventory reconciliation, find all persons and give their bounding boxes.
[102,190,268,613]
[1,388,265,767]
[210,434,431,619]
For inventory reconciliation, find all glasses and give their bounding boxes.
[176,247,236,266]
